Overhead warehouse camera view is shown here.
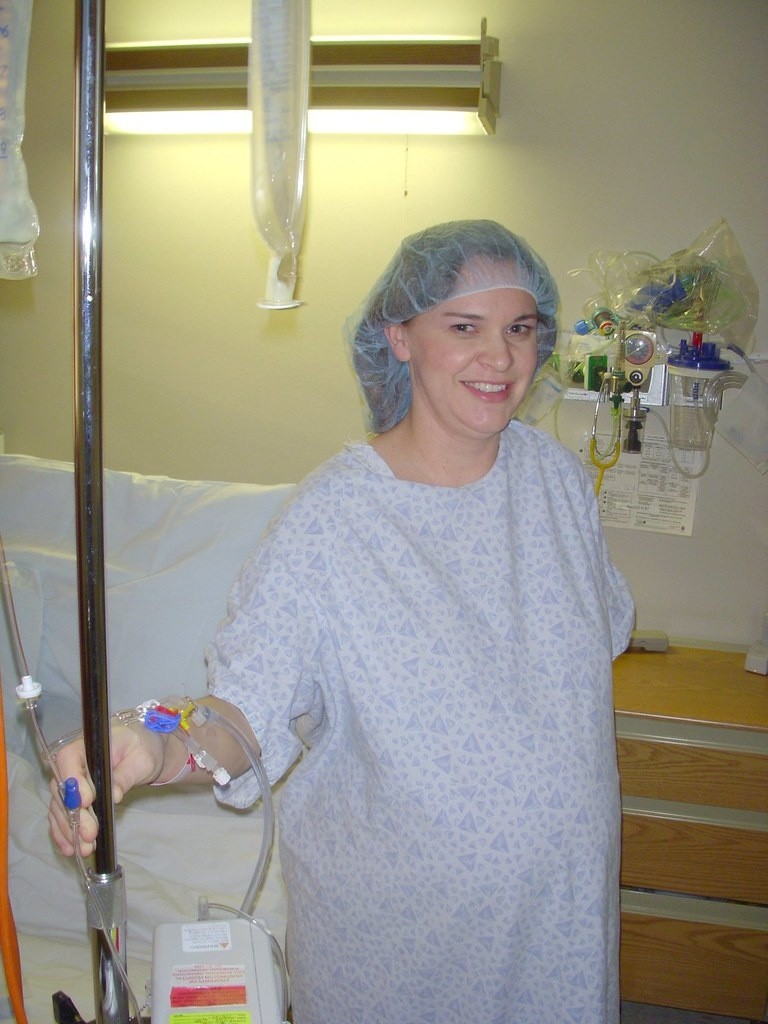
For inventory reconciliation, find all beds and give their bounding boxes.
[0,452,304,1024]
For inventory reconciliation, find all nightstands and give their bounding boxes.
[607,652,768,1021]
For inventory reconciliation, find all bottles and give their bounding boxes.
[667,373,722,451]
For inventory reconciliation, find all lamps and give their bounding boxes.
[83,16,497,140]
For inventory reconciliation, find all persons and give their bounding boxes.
[48,221,637,1024]
